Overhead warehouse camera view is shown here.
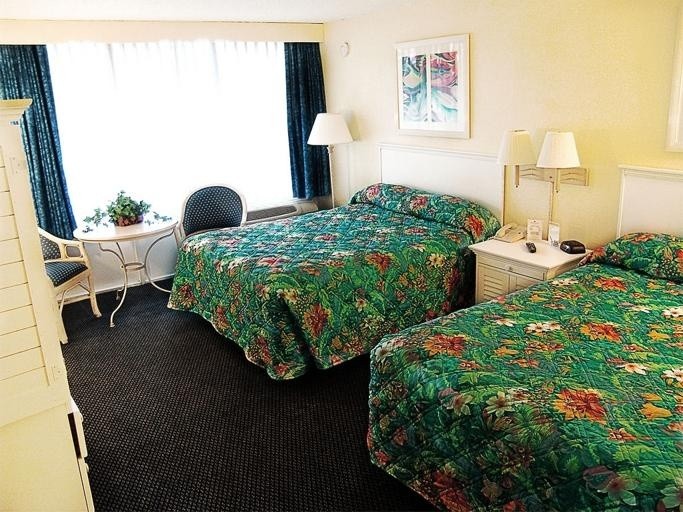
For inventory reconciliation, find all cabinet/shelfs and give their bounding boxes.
[0,96,98,512]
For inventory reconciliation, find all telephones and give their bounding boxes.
[494,223,527,242]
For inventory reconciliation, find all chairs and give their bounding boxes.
[37,184,247,345]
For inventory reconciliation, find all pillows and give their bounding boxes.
[347,181,502,241]
[579,231,683,283]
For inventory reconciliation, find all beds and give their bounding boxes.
[166,143,507,380]
[363,167,683,512]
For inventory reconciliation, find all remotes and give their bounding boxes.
[526,241,537,254]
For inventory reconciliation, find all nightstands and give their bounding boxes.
[469,235,594,305]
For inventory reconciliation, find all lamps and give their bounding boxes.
[307,113,354,208]
[493,128,589,193]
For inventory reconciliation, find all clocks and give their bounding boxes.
[338,43,350,57]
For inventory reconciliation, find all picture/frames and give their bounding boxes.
[396,33,470,139]
